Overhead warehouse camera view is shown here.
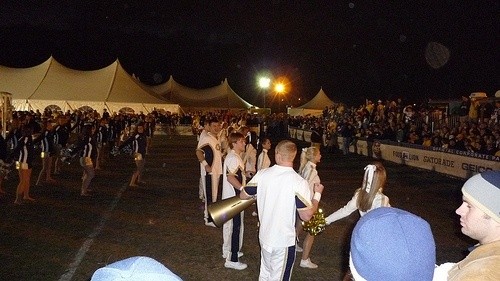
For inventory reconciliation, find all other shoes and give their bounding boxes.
[205,221,216,227]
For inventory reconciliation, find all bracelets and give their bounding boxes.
[239,186,244,191]
[312,191,321,202]
[201,159,208,167]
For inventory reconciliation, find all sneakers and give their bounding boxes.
[221,251,245,258]
[300,257,318,268]
[296,245,303,252]
[224,258,248,270]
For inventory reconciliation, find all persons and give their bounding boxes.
[239,140,324,281]
[221,132,248,270]
[349,207,436,281]
[447,170,500,281]
[295,146,322,269]
[324,162,392,225]
[0,96,500,226]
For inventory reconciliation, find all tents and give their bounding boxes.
[0,53,340,117]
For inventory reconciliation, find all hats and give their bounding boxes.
[91,255,182,281]
[461,171,500,221]
[349,207,435,281]
